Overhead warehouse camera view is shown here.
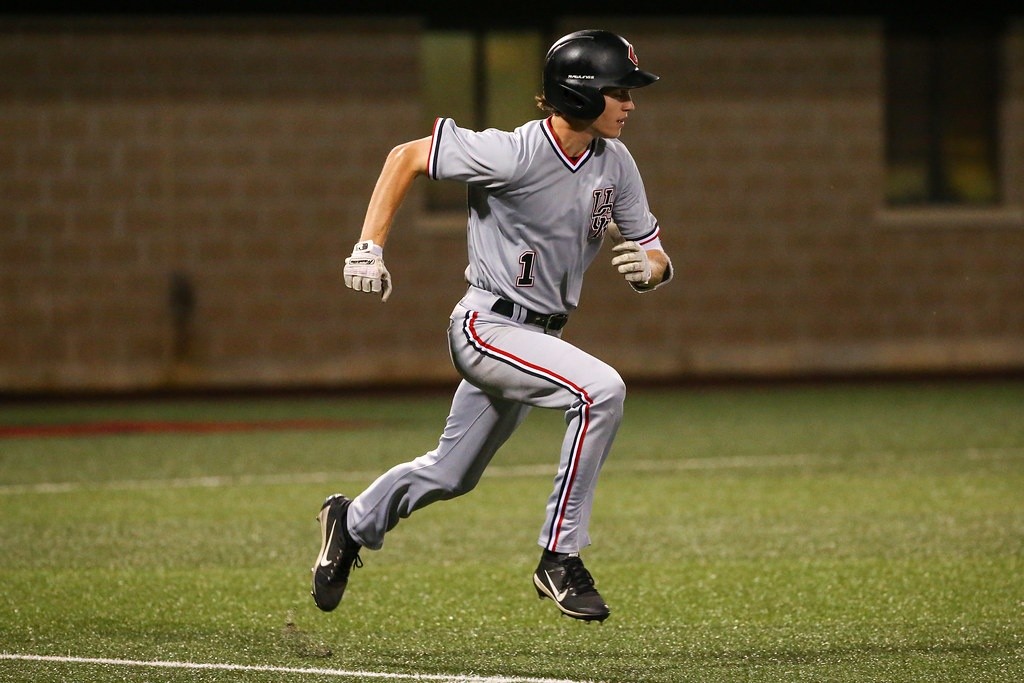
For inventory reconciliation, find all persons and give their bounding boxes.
[313,28,674,623]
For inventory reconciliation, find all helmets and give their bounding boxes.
[543,29,660,122]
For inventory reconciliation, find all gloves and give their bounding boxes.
[607,222,652,285]
[343,240,392,302]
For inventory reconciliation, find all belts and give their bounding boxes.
[491,298,568,335]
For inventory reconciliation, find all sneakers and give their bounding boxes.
[311,494,363,612]
[534,552,610,622]
